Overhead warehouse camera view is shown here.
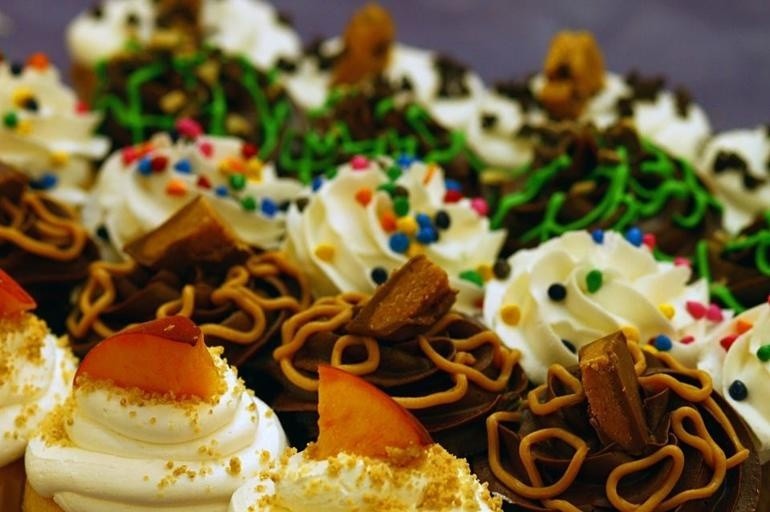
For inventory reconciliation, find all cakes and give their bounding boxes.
[0,0,770,512]
